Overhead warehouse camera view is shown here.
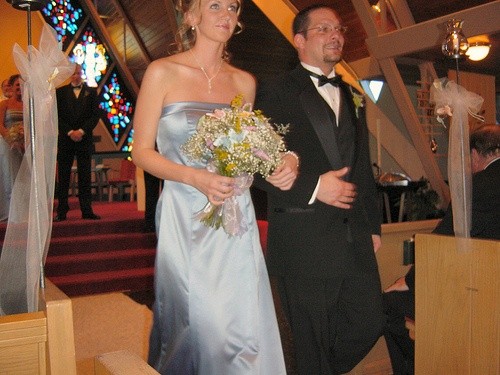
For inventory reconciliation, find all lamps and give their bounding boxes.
[466,35,492,62]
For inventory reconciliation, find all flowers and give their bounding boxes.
[5,122,25,156]
[350,85,365,119]
[180,93,290,235]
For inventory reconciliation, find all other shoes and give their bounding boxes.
[82,212,100,219]
[54,215,66,221]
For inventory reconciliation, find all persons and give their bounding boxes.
[383,124,500,375]
[52,63,101,221]
[0,75,25,222]
[133,0,300,375]
[251,5,385,375]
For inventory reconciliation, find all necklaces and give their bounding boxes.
[190,48,223,93]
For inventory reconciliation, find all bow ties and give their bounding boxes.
[302,67,343,89]
[72,85,81,89]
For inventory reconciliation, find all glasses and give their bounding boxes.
[298,24,348,36]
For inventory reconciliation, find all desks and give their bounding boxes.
[68,166,111,201]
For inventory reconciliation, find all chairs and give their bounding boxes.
[108,161,135,204]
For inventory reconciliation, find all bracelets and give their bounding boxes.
[285,150,299,167]
